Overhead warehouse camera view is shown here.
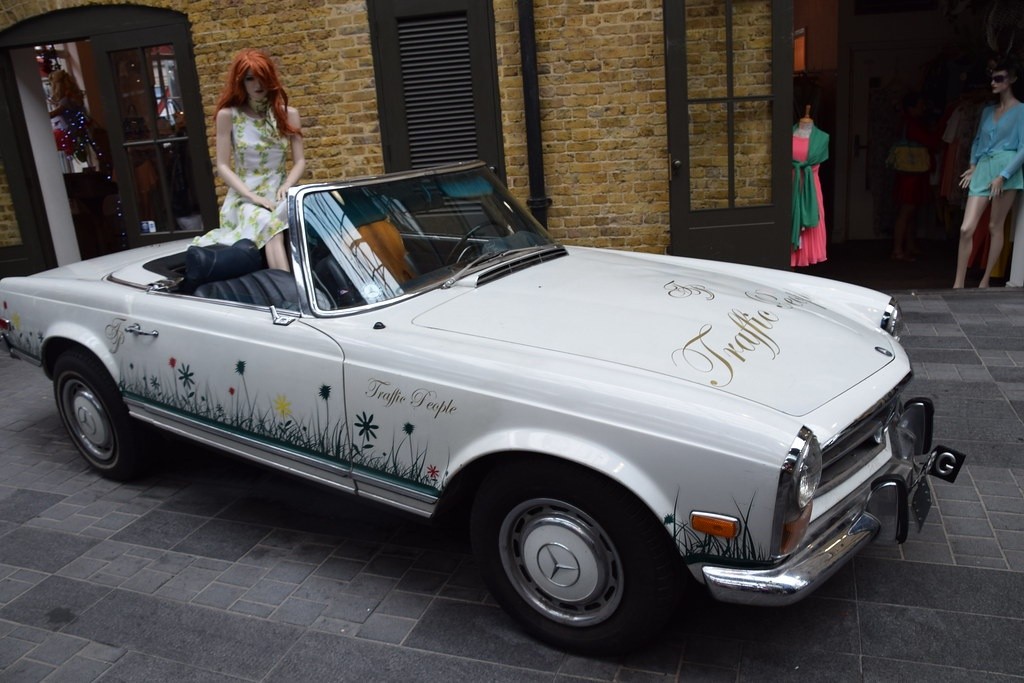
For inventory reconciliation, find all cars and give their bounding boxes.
[1,156,969,652]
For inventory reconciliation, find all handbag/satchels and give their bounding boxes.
[124,105,150,141]
[156,115,173,137]
[885,118,931,173]
[171,99,187,137]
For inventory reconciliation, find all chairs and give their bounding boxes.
[185,237,331,316]
[341,197,420,283]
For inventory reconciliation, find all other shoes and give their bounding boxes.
[890,250,916,262]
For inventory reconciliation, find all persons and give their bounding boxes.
[890,90,938,262]
[212,46,307,272]
[48,69,99,173]
[791,117,829,266]
[953,61,1024,288]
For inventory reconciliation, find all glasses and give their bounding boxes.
[989,74,1008,82]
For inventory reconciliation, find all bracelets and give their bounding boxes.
[1001,175,1007,182]
